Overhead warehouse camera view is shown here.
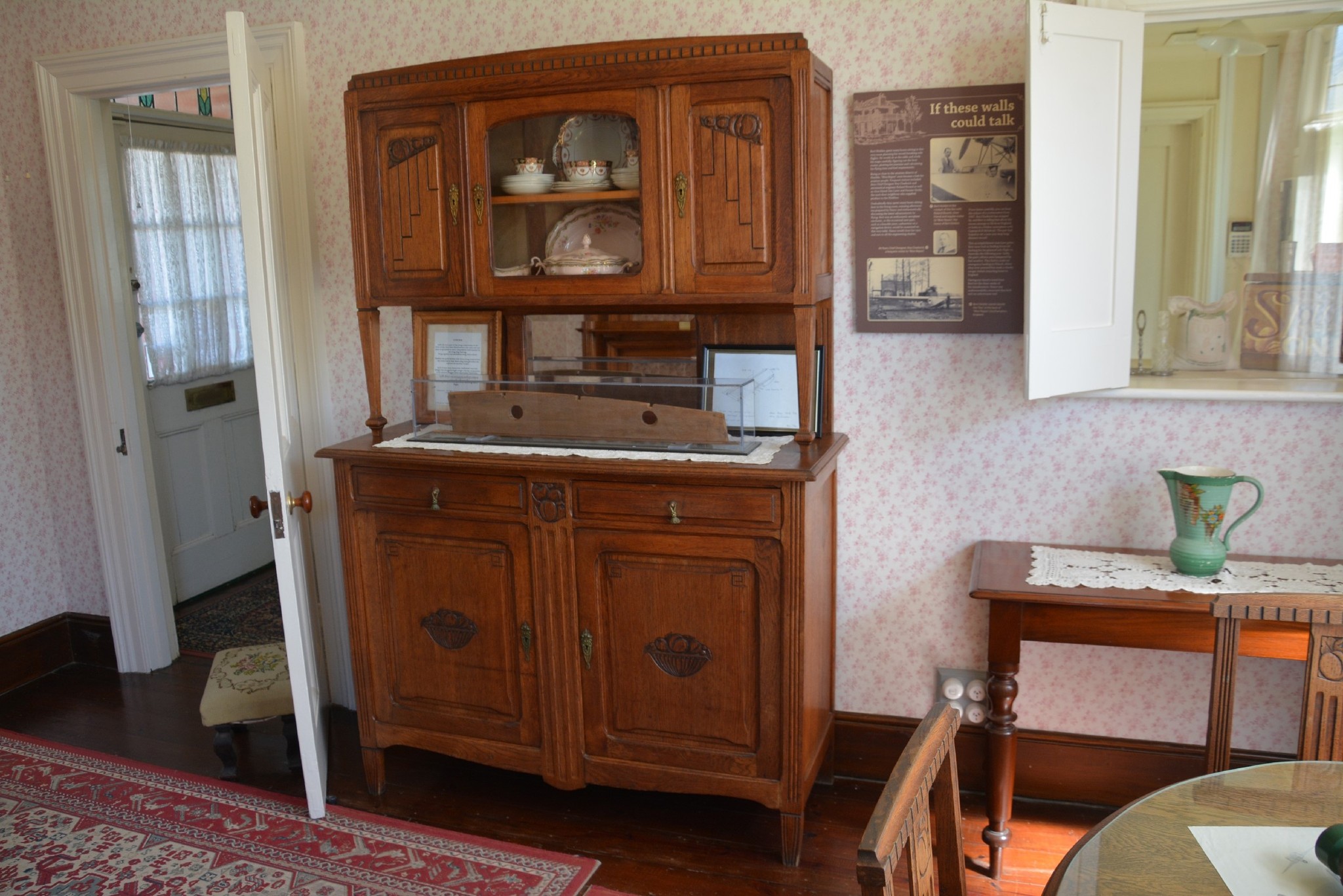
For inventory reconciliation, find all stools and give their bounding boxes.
[200,642,302,781]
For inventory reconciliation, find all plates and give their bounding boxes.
[550,180,612,193]
[499,174,555,195]
[555,111,640,182]
[610,168,639,190]
[544,202,642,272]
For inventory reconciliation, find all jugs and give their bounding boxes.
[1157,465,1264,577]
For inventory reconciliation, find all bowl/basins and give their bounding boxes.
[514,164,544,174]
[511,157,546,164]
[534,233,640,275]
[564,160,612,182]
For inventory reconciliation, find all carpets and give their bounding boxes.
[175,572,285,659]
[0,729,601,896]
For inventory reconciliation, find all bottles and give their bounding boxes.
[1152,310,1174,376]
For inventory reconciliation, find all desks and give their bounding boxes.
[969,540,1343,896]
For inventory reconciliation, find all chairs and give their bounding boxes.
[858,702,966,896]
[1206,593,1343,773]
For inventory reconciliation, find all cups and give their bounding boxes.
[1280,240,1297,274]
[626,151,639,168]
[494,256,542,276]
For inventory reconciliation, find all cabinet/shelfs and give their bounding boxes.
[314,32,850,866]
